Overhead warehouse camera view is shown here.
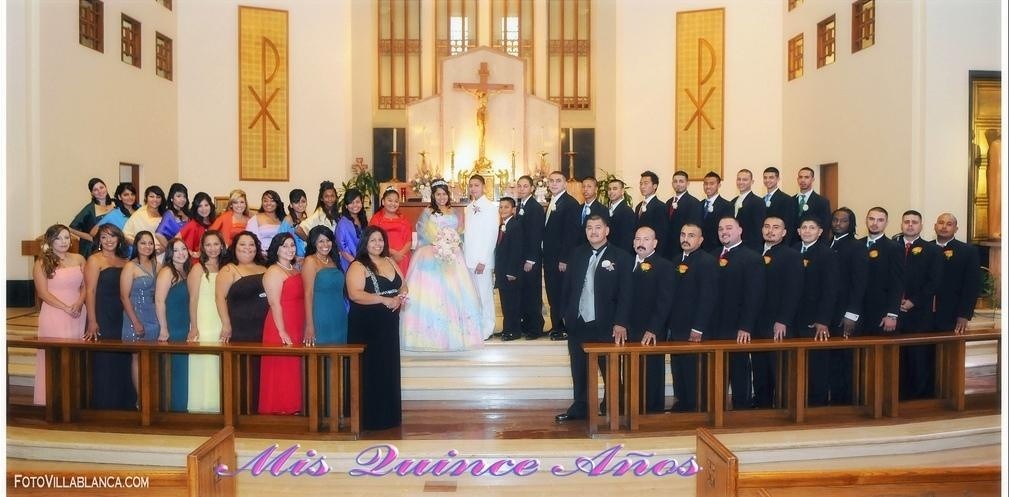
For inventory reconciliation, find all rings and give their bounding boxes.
[480,270,482,273]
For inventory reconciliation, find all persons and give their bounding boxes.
[462,174,499,341]
[400,178,485,353]
[460,85,506,147]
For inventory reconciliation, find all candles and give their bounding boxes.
[450,126,455,152]
[569,126,574,152]
[512,128,515,152]
[393,128,397,152]
[541,126,544,151]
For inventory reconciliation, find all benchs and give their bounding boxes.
[7,425,237,497]
[693,422,1000,496]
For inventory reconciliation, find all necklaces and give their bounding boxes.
[437,205,448,212]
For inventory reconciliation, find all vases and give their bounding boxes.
[534,186,548,203]
[419,186,432,203]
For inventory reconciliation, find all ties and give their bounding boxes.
[799,195,806,216]
[803,247,807,253]
[639,202,647,218]
[704,201,711,220]
[497,224,505,245]
[583,206,590,224]
[906,243,912,258]
[587,250,600,263]
[869,241,873,248]
[669,197,678,220]
[721,249,728,258]
[766,194,771,204]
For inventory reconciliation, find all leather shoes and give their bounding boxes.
[555,410,586,422]
[494,329,568,340]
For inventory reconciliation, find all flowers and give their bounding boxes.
[802,246,955,267]
[409,161,443,195]
[434,230,463,263]
[517,200,810,216]
[531,170,549,188]
[599,256,774,273]
[471,204,481,216]
[501,225,507,232]
